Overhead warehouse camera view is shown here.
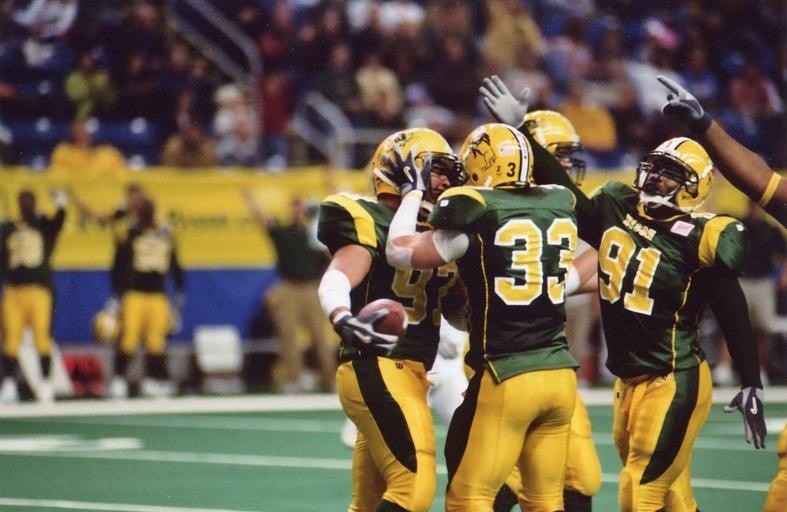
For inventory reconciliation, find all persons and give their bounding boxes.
[315,76,787,511]
[1,0,787,404]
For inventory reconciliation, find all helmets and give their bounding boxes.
[459,123,534,189]
[370,128,458,193]
[639,136,714,214]
[516,110,581,156]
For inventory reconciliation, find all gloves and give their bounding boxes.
[656,75,711,134]
[478,75,532,130]
[332,309,399,360]
[724,387,767,449]
[373,144,427,196]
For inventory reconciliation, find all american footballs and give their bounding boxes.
[358,298,408,334]
